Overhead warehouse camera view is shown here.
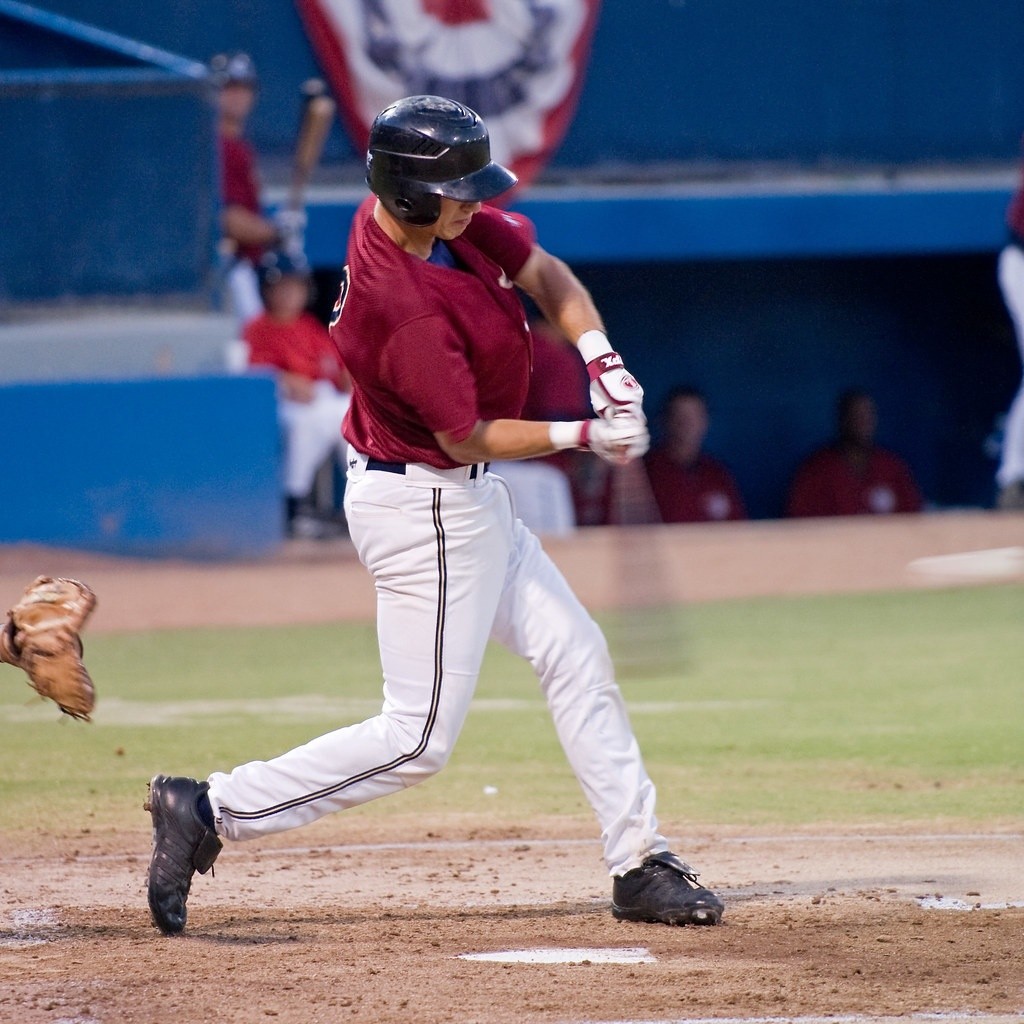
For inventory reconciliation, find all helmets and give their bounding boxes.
[366,92,519,226]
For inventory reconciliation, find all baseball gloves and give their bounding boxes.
[0,572,106,724]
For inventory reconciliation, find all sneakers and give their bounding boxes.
[146,774,224,933]
[613,854,725,926]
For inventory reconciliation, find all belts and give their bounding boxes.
[367,453,491,480]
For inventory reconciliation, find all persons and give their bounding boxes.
[3,571,102,722]
[217,79,1024,543]
[146,93,742,936]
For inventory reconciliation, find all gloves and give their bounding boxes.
[578,331,644,419]
[550,413,651,465]
